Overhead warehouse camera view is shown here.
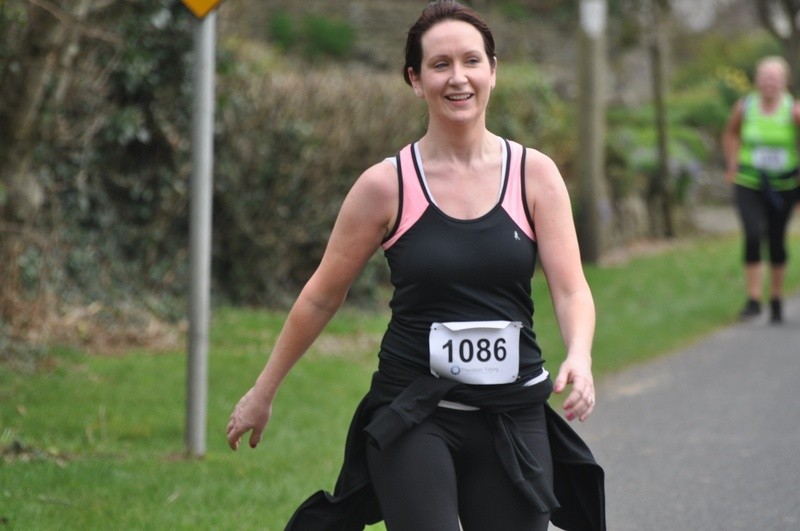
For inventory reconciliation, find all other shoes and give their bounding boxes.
[770,298,781,322]
[740,299,762,318]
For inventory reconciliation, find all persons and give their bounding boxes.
[227,0,605,530]
[723,57,800,324]
